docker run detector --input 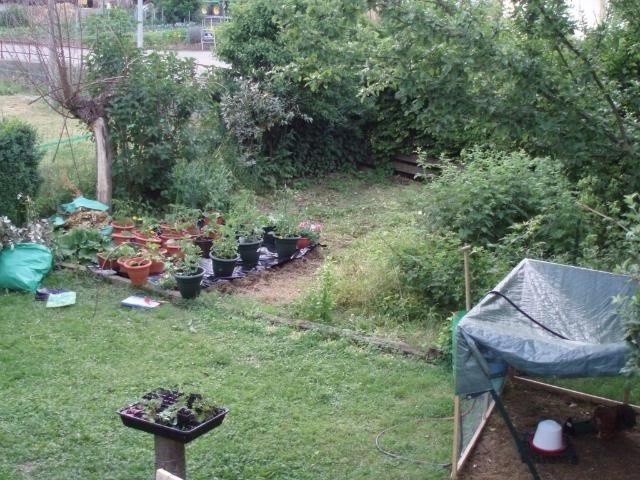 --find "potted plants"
[96,196,324,299]
